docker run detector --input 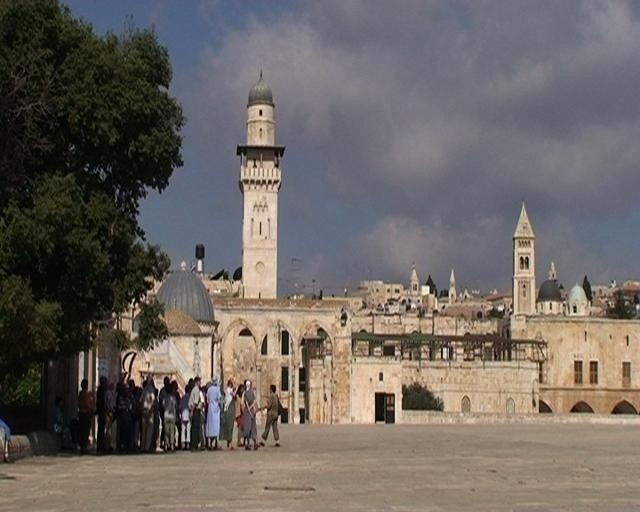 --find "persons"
[0,419,10,463]
[52,372,280,453]
